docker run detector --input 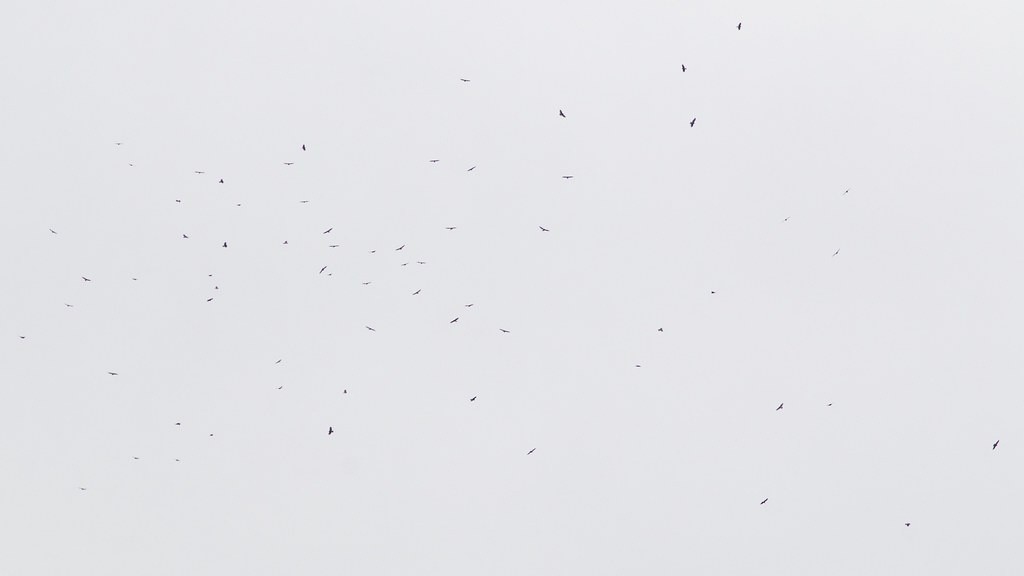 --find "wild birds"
[15,18,999,552]
